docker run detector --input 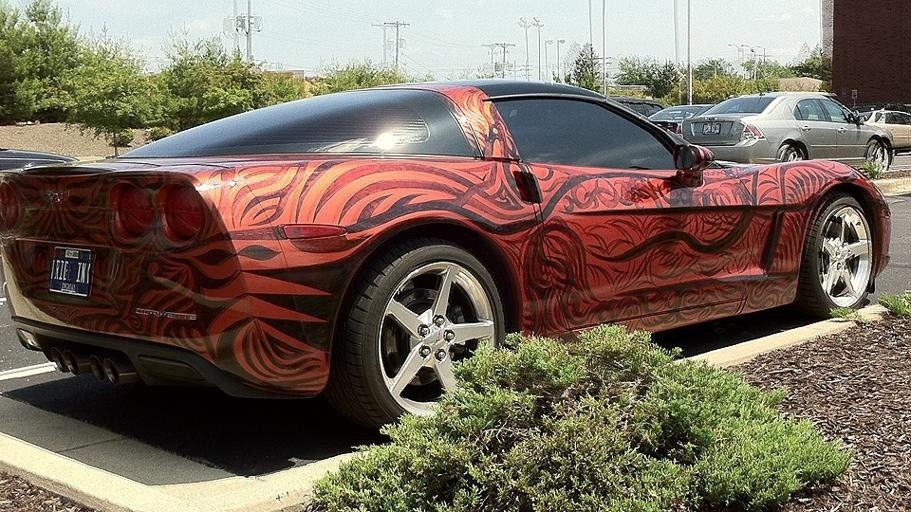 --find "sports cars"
[0,78,893,432]
